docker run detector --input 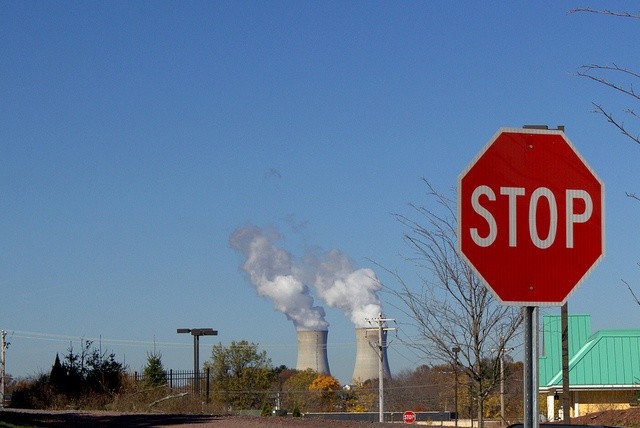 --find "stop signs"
[458,128,605,306]
[402,411,415,424]
